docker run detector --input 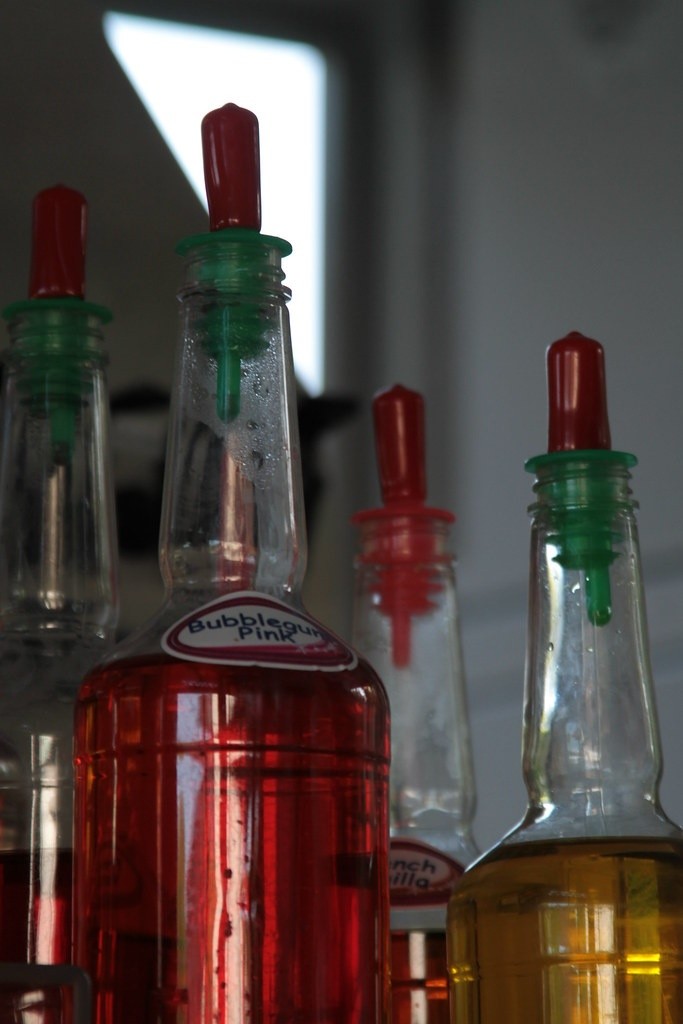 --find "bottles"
[446,331,681,1024]
[350,381,483,1024]
[72,102,393,1024]
[0,182,117,1024]
